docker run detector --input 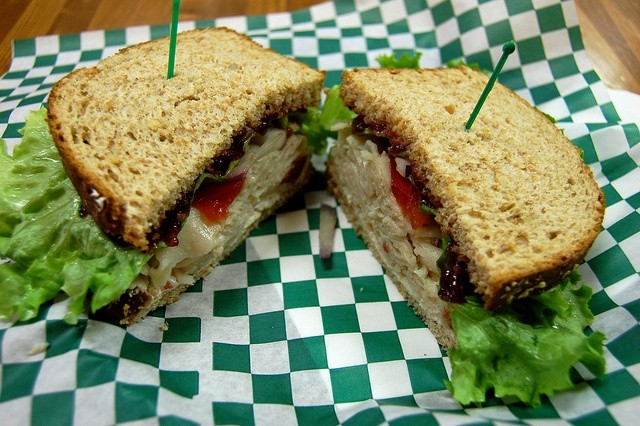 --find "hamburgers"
[0,25,325,325]
[324,66,607,408]
